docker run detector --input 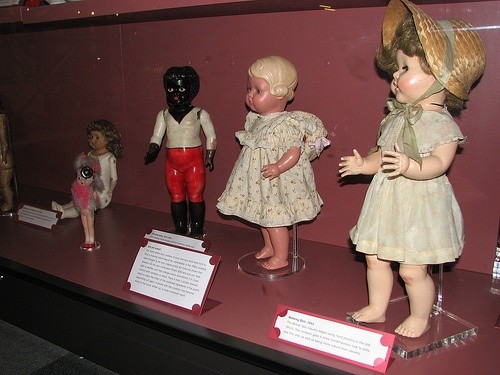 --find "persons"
[216,55,331,271]
[50,119,122,220]
[71,166,101,252]
[339,0,487,339]
[0,114,18,217]
[145,65,217,238]
